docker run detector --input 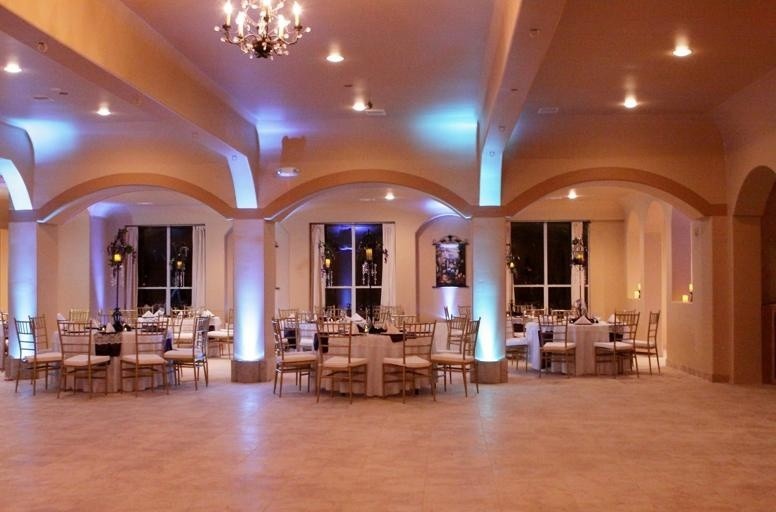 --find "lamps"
[214,1,313,64]
